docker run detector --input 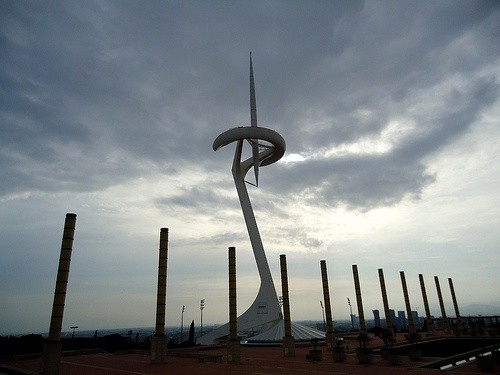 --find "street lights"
[70,326,79,340]
[199,299,206,336]
[181,305,186,335]
[347,298,355,329]
[319,301,326,332]
